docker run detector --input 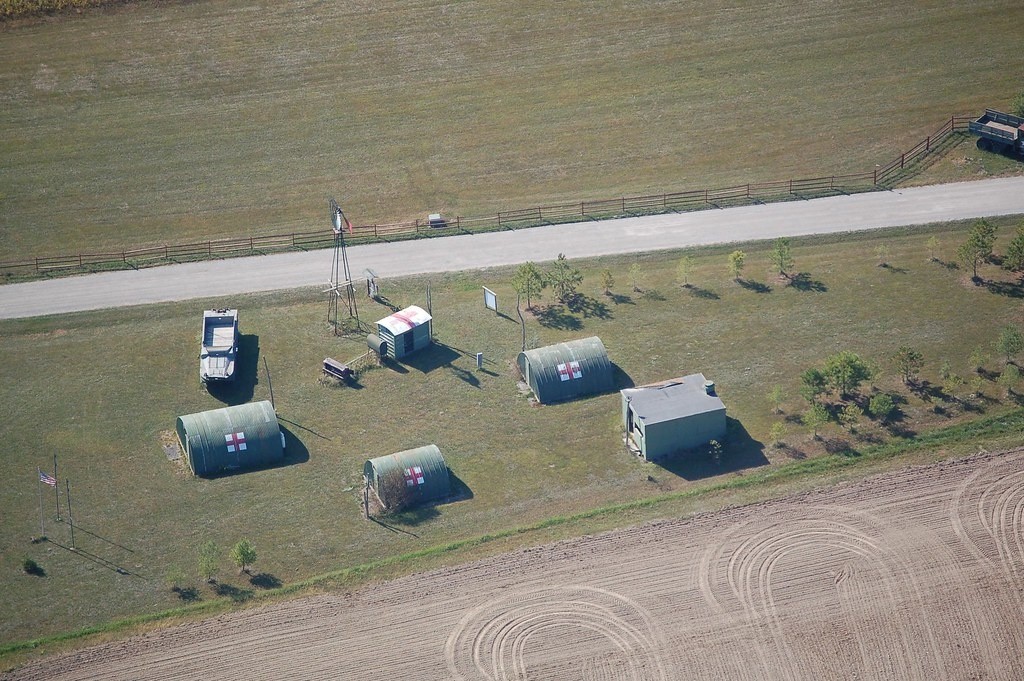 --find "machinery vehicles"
[198,307,241,386]
[967,107,1024,161]
